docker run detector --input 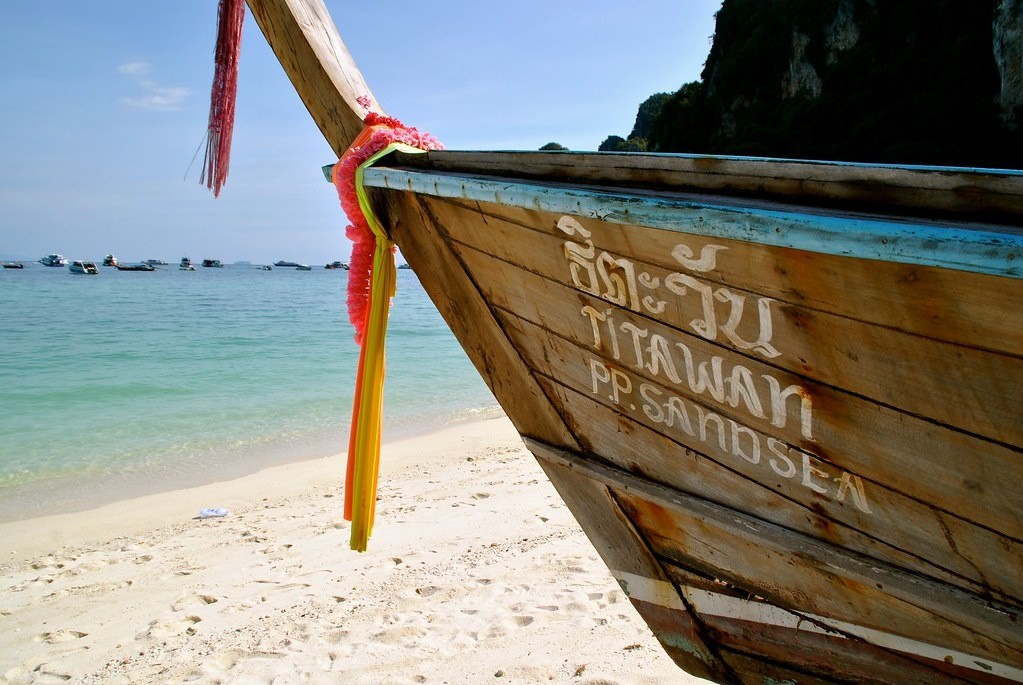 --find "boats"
[145,259,168,265]
[295,264,312,270]
[237,0,1023,684]
[115,262,155,271]
[102,254,118,266]
[398,263,410,269]
[68,260,100,274]
[255,264,273,271]
[324,260,350,271]
[178,257,195,270]
[201,259,224,268]
[273,260,299,266]
[39,254,69,267]
[1,261,24,269]
